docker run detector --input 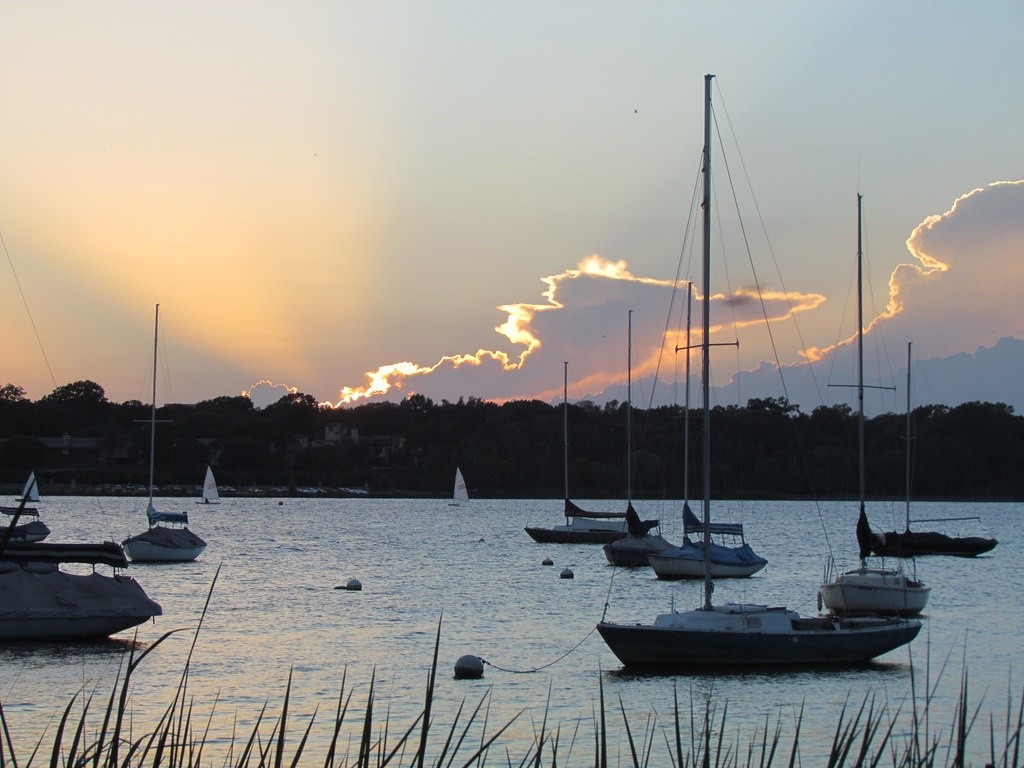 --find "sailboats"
[120,296,209,563]
[453,466,469,503]
[647,281,770,578]
[815,190,930,615]
[598,70,924,671]
[1,469,163,642]
[856,340,999,561]
[523,359,630,543]
[602,308,679,568]
[194,466,222,505]
[2,472,51,543]
